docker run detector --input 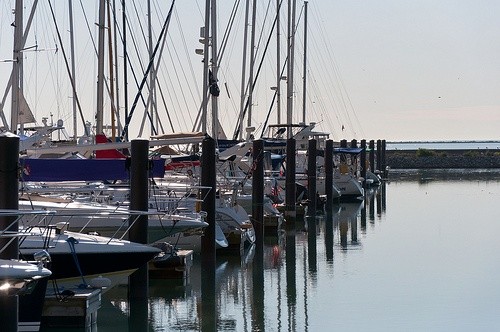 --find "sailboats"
[1,0,384,331]
